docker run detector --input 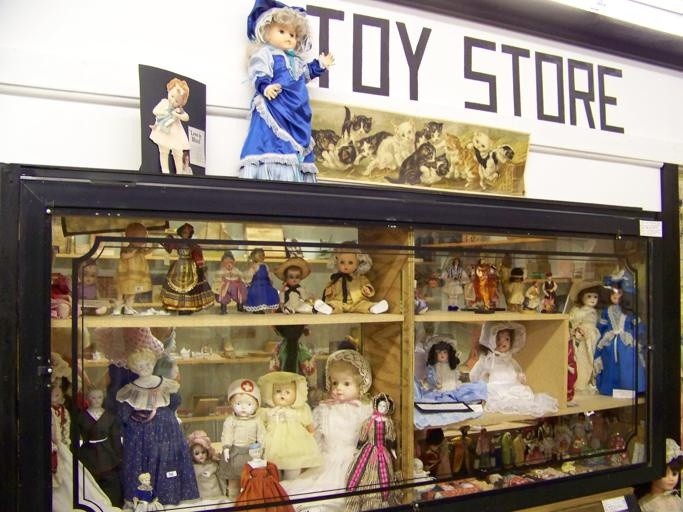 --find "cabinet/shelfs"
[16,173,666,512]
